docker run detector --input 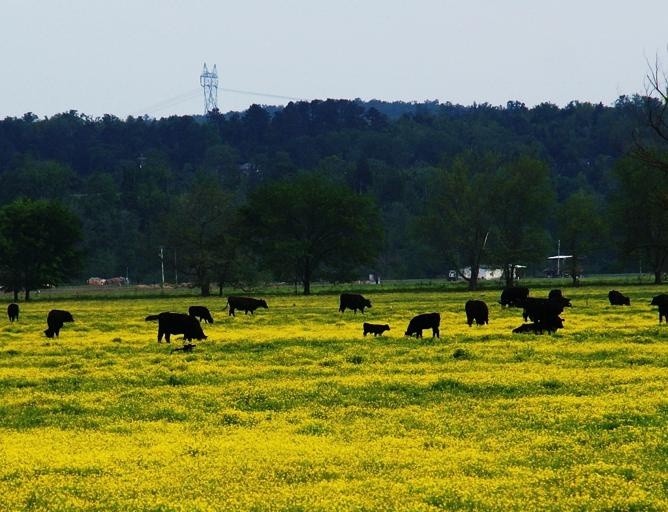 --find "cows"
[650,294,668,323]
[221,295,268,317]
[338,293,372,315]
[498,285,572,335]
[7,303,19,322]
[145,305,213,344]
[404,311,441,340]
[608,290,630,306]
[464,299,489,327]
[363,322,391,337]
[44,309,75,338]
